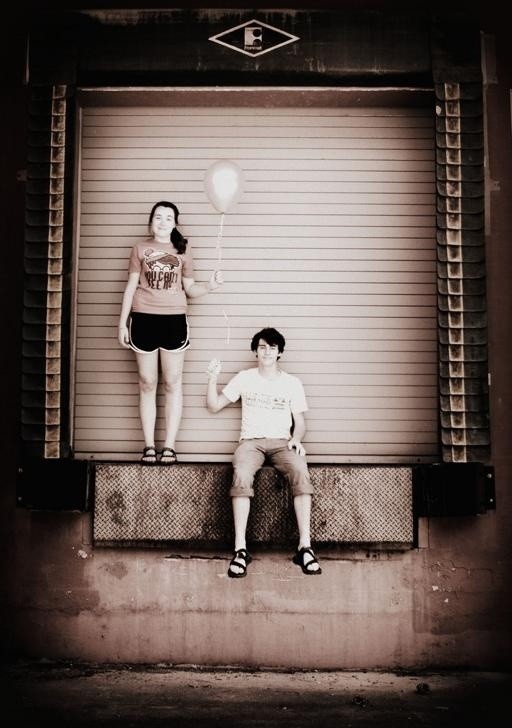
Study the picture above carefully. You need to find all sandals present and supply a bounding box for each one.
[226,548,253,579]
[291,546,322,576]
[158,446,179,465]
[140,445,158,466]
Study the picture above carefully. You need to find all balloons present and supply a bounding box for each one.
[204,160,246,216]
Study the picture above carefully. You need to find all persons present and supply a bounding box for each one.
[117,196,226,465]
[206,328,324,579]
